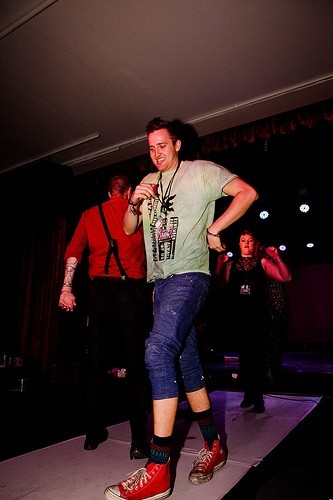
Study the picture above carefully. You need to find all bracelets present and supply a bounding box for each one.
[276,260,282,265]
[128,198,142,216]
[207,228,220,238]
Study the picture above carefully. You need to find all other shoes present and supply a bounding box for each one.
[84,431,108,450]
[130,445,151,460]
[247,407,265,414]
[240,400,253,408]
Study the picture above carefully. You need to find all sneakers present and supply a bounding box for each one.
[104,457,170,500]
[188,435,225,485]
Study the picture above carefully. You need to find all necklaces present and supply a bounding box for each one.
[240,259,254,295]
[155,162,182,240]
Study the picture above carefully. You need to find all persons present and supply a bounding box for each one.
[59,175,153,460]
[104,120,258,500]
[212,230,292,414]
